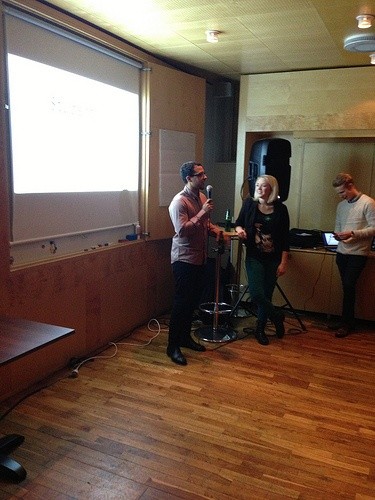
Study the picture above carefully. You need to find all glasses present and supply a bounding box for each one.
[190,172,207,177]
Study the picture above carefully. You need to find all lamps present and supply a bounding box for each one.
[356,14,374,29]
[205,30,220,43]
[369,53,375,64]
[344,33,375,53]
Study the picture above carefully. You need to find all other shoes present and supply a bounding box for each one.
[335,322,357,337]
[269,306,284,337]
[328,318,345,329]
[255,329,269,345]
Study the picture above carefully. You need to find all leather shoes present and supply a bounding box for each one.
[179,335,206,352]
[167,344,186,365]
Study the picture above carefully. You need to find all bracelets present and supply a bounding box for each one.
[351,231,354,237]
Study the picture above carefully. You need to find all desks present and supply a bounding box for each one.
[0,314,78,482]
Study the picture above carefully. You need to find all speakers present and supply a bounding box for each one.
[249,138,292,202]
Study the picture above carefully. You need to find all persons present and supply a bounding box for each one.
[166,162,226,366]
[234,175,290,345]
[332,173,375,337]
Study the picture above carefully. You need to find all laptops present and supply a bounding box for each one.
[322,231,339,252]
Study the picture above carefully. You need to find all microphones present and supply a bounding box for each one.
[206,185,213,218]
